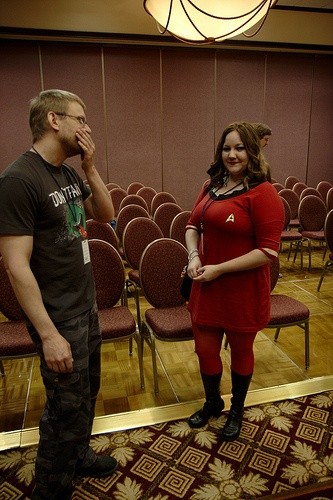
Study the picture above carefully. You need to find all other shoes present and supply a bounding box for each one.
[75,454,118,477]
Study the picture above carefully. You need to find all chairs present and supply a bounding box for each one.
[0,176,333,396]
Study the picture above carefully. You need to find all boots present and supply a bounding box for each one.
[220,368,253,441]
[189,370,223,428]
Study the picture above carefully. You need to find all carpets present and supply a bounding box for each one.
[0,390,333,500]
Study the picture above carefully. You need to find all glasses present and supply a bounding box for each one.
[55,112,89,128]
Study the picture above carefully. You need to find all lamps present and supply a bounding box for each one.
[143,0,279,46]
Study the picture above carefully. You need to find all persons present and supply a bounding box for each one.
[250,122,272,183]
[0,89,119,500]
[184,121,285,441]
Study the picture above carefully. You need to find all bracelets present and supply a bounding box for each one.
[187,249,199,263]
